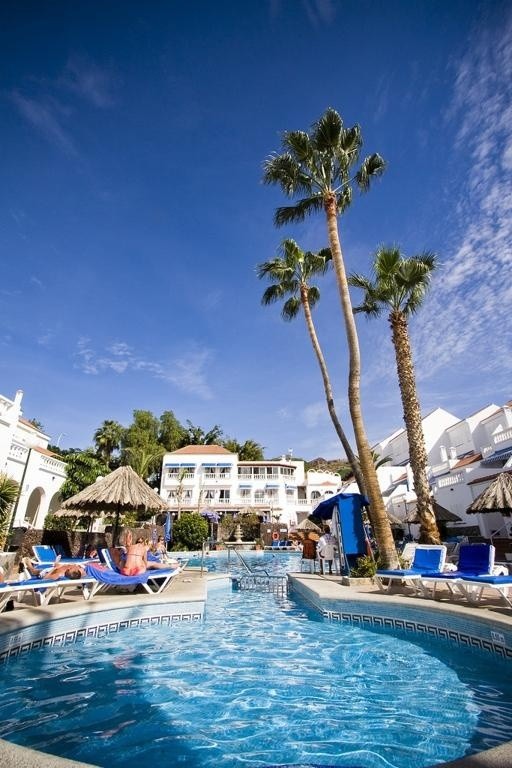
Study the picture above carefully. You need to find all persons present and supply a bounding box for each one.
[316,525,339,574]
[120,536,147,574]
[26,554,87,580]
[155,535,177,564]
[144,538,181,570]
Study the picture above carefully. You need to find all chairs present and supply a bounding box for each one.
[372,536,512,608]
[263,540,301,550]
[0,545,190,615]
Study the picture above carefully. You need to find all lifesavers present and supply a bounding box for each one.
[272,532,279,541]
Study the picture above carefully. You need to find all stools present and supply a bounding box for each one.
[300,557,338,575]
